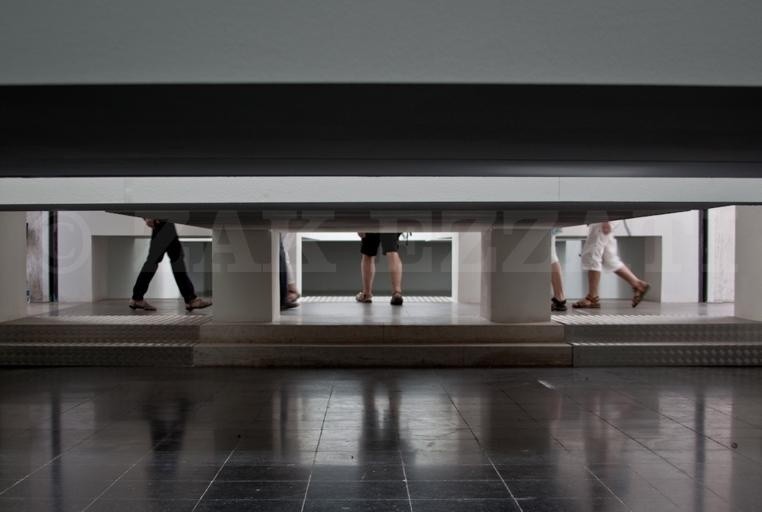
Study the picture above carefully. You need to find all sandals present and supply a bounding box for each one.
[572,295,599,308]
[391,292,402,305]
[551,298,567,311]
[357,292,372,302]
[632,280,650,308]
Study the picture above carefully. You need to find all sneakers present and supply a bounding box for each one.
[280,290,300,311]
[129,301,157,311]
[186,299,212,312]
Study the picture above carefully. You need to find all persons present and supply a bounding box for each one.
[128,215,213,312]
[280,231,301,311]
[280,231,300,302]
[551,232,570,312]
[571,218,652,309]
[354,230,407,304]
